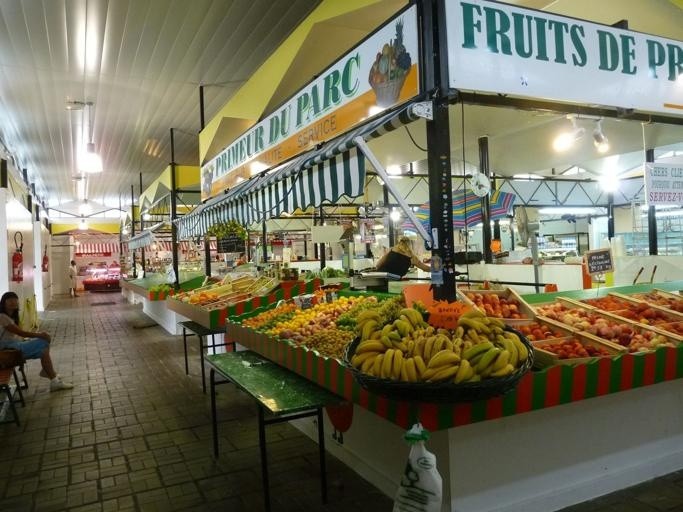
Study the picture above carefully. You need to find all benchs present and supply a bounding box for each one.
[0,351,33,432]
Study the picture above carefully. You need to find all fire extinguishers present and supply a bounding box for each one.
[11,231,23,281]
[42,244,49,272]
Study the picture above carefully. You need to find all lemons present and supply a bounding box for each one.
[382,43,390,56]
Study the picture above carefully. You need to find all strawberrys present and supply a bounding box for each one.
[397,49,412,70]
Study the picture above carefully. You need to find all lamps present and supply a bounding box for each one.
[588,115,612,157]
[547,115,583,150]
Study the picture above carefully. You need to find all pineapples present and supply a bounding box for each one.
[394,44,406,58]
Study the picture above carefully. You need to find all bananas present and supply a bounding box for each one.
[355,308,529,385]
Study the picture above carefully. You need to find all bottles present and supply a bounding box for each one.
[561,237,575,248]
[537,236,548,249]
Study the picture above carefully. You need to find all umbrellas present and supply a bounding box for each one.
[402,189,517,232]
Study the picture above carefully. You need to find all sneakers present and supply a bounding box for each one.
[50,377,74,392]
[38,368,61,380]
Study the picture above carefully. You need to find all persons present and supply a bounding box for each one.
[0,292,73,392]
[68,260,80,298]
[110,261,120,267]
[237,253,247,266]
[375,235,431,277]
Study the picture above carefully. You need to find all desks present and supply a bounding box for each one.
[175,317,226,395]
[198,348,343,511]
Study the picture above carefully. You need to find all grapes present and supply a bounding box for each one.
[305,294,406,360]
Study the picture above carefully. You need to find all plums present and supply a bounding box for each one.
[467,292,683,360]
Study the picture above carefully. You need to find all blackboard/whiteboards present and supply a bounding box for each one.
[584,247,615,276]
[217,229,245,253]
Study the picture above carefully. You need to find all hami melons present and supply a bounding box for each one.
[378,56,389,75]
